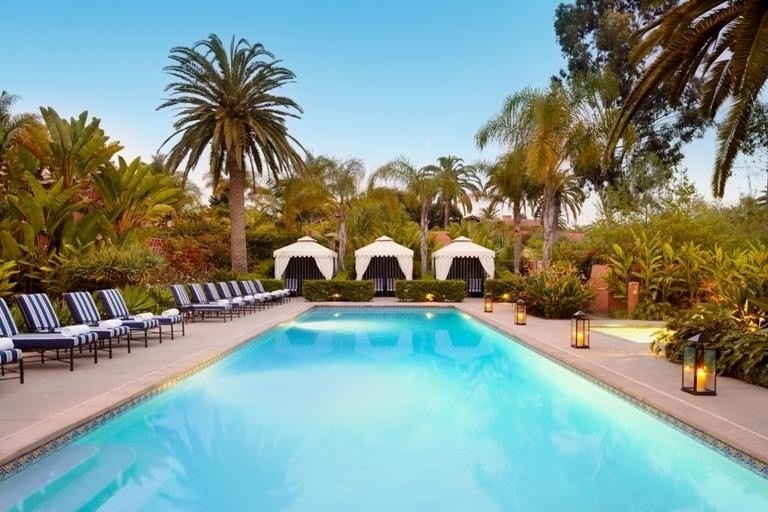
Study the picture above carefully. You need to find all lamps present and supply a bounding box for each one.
[484,292,493,312]
[570,309,590,349]
[681,333,718,396]
[514,298,527,325]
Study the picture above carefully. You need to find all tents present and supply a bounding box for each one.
[353,235,414,296]
[431,236,496,298]
[269,234,337,294]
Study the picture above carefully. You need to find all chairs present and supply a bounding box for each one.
[286,278,298,292]
[17,293,130,359]
[98,289,185,340]
[0,298,99,371]
[387,278,398,291]
[0,349,24,384]
[469,278,482,294]
[371,277,383,292]
[169,280,290,325]
[63,291,162,349]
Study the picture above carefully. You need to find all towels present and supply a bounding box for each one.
[0,337,14,351]
[61,324,91,337]
[134,313,154,322]
[162,307,180,317]
[97,318,123,329]
